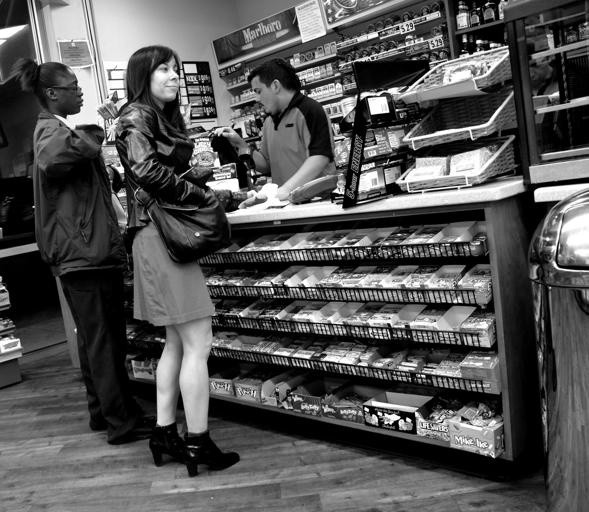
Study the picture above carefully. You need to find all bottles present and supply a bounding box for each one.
[566,26,578,44]
[456,0,506,56]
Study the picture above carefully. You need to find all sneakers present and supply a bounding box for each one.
[110,415,155,445]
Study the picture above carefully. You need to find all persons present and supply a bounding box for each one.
[209,56,338,201]
[115,43,242,478]
[12,55,159,446]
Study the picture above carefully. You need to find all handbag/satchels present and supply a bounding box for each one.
[150,190,232,264]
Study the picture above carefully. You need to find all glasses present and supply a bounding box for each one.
[51,85,78,92]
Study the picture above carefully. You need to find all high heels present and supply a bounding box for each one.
[150,423,185,466]
[183,432,240,477]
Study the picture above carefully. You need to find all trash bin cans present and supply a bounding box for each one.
[528,188,589,512]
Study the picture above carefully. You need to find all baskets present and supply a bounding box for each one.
[395,132,516,193]
[402,89,516,149]
[402,44,512,106]
